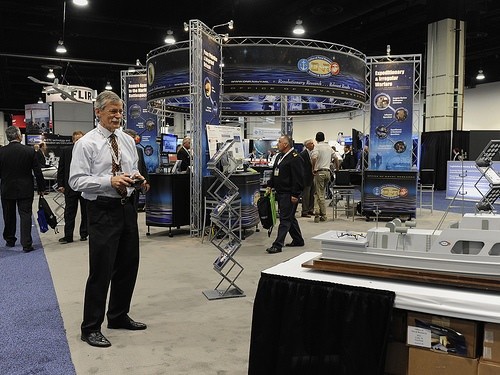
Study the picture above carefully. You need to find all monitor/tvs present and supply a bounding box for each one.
[161,133,178,153]
[25,134,43,146]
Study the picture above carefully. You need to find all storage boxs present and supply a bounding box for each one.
[387,308,500,375]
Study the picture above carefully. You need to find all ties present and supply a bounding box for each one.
[108,134,127,198]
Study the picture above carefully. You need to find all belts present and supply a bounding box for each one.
[87,198,133,205]
[318,169,330,171]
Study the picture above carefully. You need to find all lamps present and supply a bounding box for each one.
[183,22,189,32]
[164,30,176,44]
[212,20,234,31]
[46,68,55,79]
[55,1,67,54]
[475,70,484,80]
[105,81,112,90]
[218,33,229,41]
[293,19,305,35]
[37,97,43,103]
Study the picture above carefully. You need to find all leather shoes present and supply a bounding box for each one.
[81,331,112,347]
[107,318,147,330]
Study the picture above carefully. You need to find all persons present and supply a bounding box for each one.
[0,126,46,252]
[33,142,50,168]
[124,129,149,210]
[266,151,273,161]
[57,130,88,243]
[360,146,368,170]
[326,145,358,199]
[310,132,338,222]
[68,90,147,348]
[453,148,461,161]
[177,138,191,171]
[300,139,315,218]
[265,134,307,252]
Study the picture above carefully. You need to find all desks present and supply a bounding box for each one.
[144,170,261,237]
[246,251,500,375]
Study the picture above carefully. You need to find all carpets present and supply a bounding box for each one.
[1,204,77,375]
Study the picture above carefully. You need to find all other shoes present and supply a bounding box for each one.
[5,243,15,247]
[320,216,326,221]
[285,241,296,247]
[308,211,315,215]
[23,247,34,253]
[266,246,282,253]
[301,213,311,218]
[79,237,87,241]
[314,217,320,223]
[59,236,73,243]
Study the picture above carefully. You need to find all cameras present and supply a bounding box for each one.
[130,178,144,187]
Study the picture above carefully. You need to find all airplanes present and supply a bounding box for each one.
[27,76,80,102]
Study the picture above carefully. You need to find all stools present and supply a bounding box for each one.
[200,195,242,243]
[417,168,435,214]
[331,171,356,222]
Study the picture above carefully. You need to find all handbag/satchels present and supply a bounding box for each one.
[257,190,276,237]
[37,195,49,233]
[40,195,58,229]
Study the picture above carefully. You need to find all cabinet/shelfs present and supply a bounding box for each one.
[201,139,245,300]
